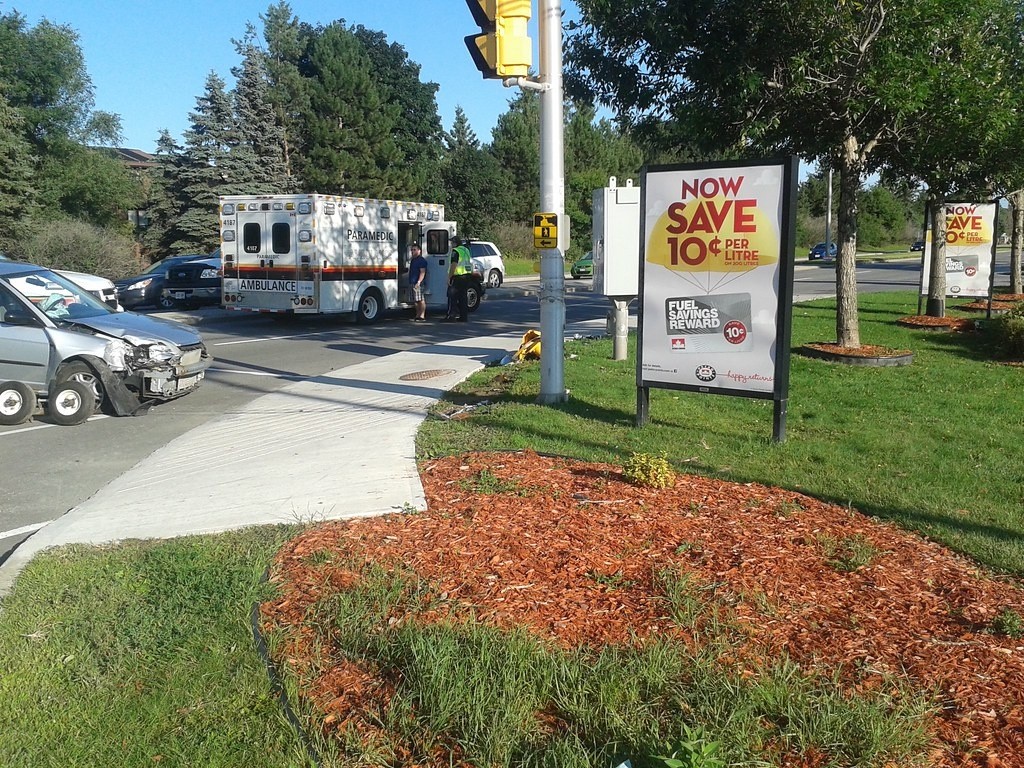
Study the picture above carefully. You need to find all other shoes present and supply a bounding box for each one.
[415,317,426,322]
[438,316,456,323]
[456,318,467,322]
[409,318,415,321]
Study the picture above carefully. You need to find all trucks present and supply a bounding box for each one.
[218,193,489,326]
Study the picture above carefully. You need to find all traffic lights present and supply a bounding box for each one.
[464,0,532,77]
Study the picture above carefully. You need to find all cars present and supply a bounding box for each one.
[110,255,210,310]
[0,258,214,427]
[458,237,505,289]
[570,250,593,280]
[809,241,837,261]
[910,241,924,252]
[0,254,124,315]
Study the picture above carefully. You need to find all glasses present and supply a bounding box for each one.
[411,249,419,251]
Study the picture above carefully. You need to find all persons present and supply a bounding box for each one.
[440,236,473,322]
[406,244,428,322]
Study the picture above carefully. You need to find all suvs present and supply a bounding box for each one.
[166,246,221,311]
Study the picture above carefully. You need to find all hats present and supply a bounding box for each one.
[448,236,462,246]
